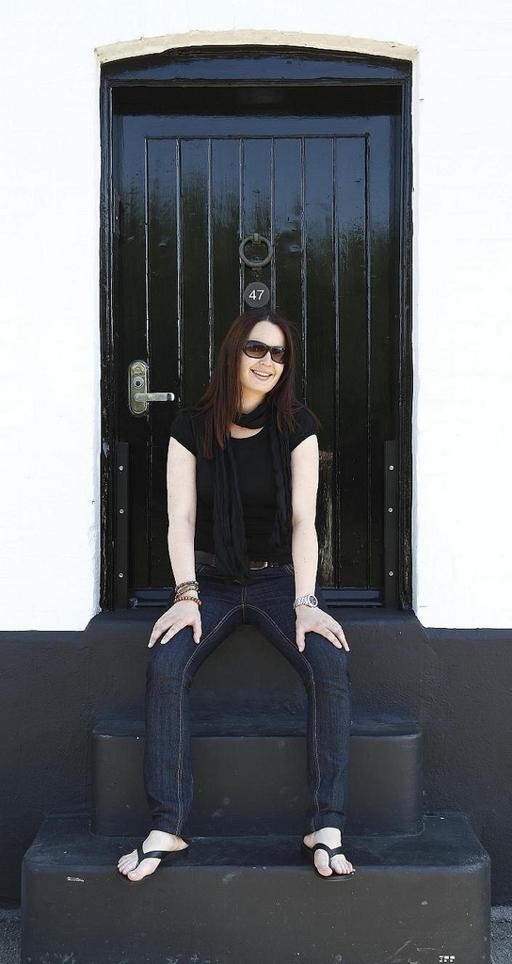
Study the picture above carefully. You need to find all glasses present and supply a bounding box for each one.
[241,340,288,364]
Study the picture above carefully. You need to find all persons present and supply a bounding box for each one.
[115,308,354,883]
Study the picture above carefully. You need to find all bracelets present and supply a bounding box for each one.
[173,581,203,605]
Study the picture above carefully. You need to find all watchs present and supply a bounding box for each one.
[293,594,318,609]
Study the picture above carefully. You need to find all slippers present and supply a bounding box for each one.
[302,836,354,883]
[113,837,191,884]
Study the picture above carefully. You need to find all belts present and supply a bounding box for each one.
[195,551,279,571]
